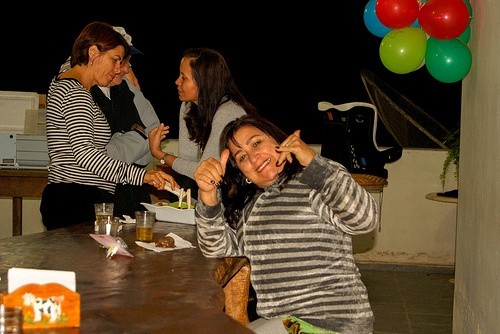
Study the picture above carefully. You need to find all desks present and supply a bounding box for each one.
[0,169,50,236]
[425,192,458,284]
[0,219,257,334]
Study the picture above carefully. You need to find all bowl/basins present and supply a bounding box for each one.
[140,202,196,226]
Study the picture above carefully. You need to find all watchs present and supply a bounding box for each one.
[160,152,169,165]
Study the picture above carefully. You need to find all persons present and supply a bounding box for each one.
[194,114,380,334]
[38,21,180,231]
[149,48,259,211]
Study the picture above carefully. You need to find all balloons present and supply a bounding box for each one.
[363,0,473,84]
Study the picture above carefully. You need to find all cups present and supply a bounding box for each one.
[98,215,119,248]
[135,212,155,241]
[94,203,115,225]
[0,307,23,334]
